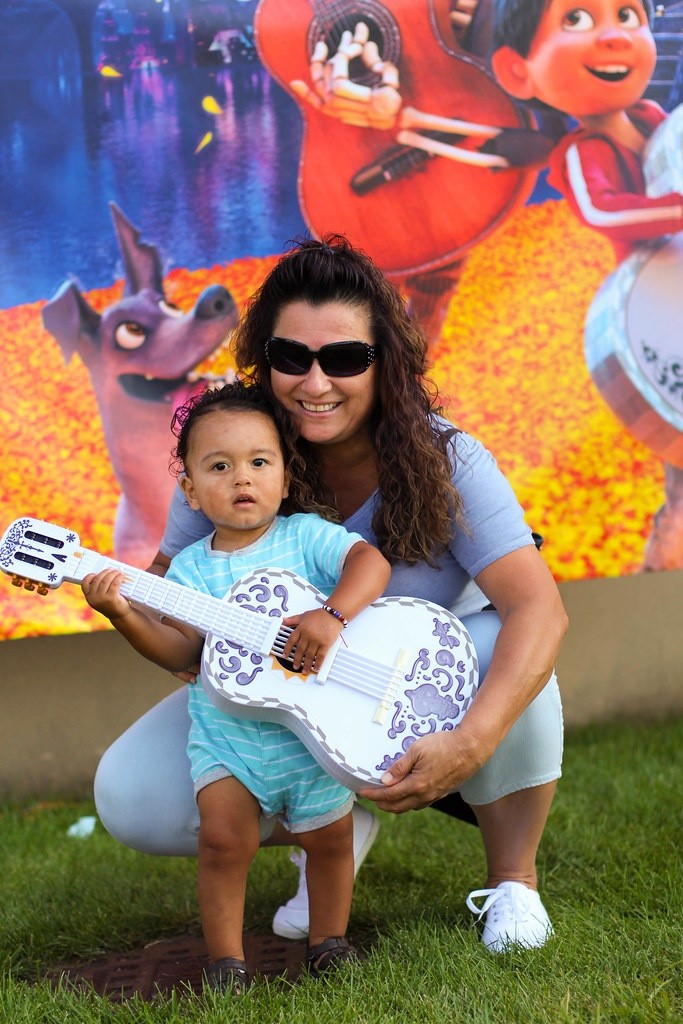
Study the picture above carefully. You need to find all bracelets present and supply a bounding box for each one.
[321,605,348,630]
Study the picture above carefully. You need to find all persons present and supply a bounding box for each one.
[94,232,569,958]
[81,381,391,996]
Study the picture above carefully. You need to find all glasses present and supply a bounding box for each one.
[264,336,378,376]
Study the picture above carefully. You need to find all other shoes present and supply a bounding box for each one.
[204,958,250,1000]
[305,939,364,983]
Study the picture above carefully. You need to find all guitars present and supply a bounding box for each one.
[1,516,481,792]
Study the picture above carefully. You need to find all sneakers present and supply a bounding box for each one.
[466,882,557,952]
[272,803,380,939]
